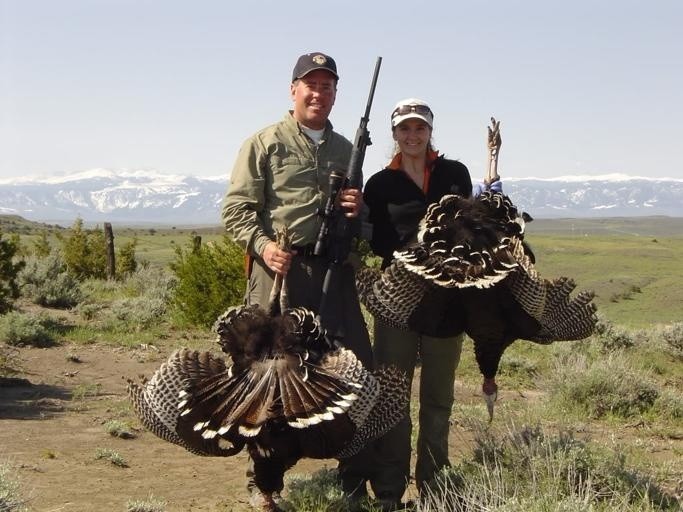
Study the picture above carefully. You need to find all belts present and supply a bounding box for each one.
[290,243,328,258]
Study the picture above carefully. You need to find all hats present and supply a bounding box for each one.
[291,52,340,82]
[391,95,435,129]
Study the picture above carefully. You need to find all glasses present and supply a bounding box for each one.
[391,103,435,123]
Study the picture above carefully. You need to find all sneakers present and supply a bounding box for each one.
[248,487,285,512]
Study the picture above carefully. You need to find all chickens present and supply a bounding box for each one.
[356,115,600,423]
[125,221,412,512]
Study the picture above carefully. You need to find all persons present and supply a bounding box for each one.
[364,99,472,512]
[221,52,372,512]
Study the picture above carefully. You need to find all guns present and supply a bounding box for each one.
[311,58,381,336]
